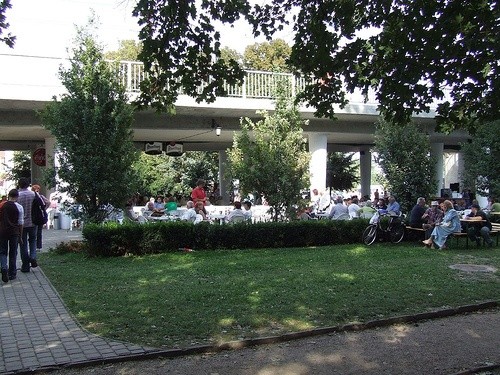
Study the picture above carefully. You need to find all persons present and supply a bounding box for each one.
[462,189,500,223]
[423,194,461,250]
[31,185,51,251]
[465,204,494,247]
[409,197,428,243]
[15,177,45,273]
[104,196,252,224]
[0,189,24,283]
[286,189,401,221]
[192,179,209,208]
[51,196,85,229]
[421,201,444,249]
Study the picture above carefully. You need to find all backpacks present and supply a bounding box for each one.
[31,192,48,225]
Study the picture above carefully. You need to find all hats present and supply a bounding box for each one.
[430,201,439,205]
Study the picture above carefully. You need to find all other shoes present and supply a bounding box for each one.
[1,268,8,282]
[21,268,30,272]
[476,237,481,247]
[487,241,493,248]
[422,239,433,244]
[438,244,447,250]
[31,256,37,268]
[9,275,16,280]
[422,223,430,230]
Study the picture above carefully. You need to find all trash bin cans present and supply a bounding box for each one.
[54,213,60,230]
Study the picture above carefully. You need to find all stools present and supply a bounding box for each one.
[491,222,500,246]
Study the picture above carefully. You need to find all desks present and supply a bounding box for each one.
[148,215,178,220]
[207,213,226,221]
[318,211,327,220]
[490,212,500,220]
[460,219,487,246]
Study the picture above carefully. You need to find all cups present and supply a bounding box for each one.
[435,220,439,225]
[422,223,427,231]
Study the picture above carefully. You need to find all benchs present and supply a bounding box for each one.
[407,227,467,249]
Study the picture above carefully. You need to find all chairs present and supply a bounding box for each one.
[136,208,352,221]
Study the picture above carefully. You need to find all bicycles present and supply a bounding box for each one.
[362,206,405,246]
